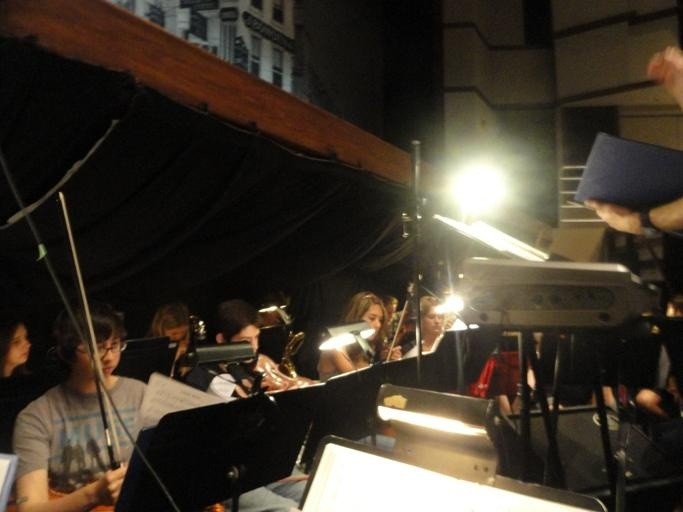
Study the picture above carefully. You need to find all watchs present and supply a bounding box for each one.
[640,208,666,242]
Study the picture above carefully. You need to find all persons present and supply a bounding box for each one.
[186,300,308,512]
[318,292,401,381]
[594,296,683,430]
[149,304,197,381]
[259,292,319,381]
[385,297,401,341]
[585,45,683,243]
[13,304,146,511]
[0,316,52,456]
[400,296,456,359]
[476,333,561,433]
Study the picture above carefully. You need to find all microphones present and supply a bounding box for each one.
[401,213,408,240]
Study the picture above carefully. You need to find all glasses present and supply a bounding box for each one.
[75,338,128,355]
